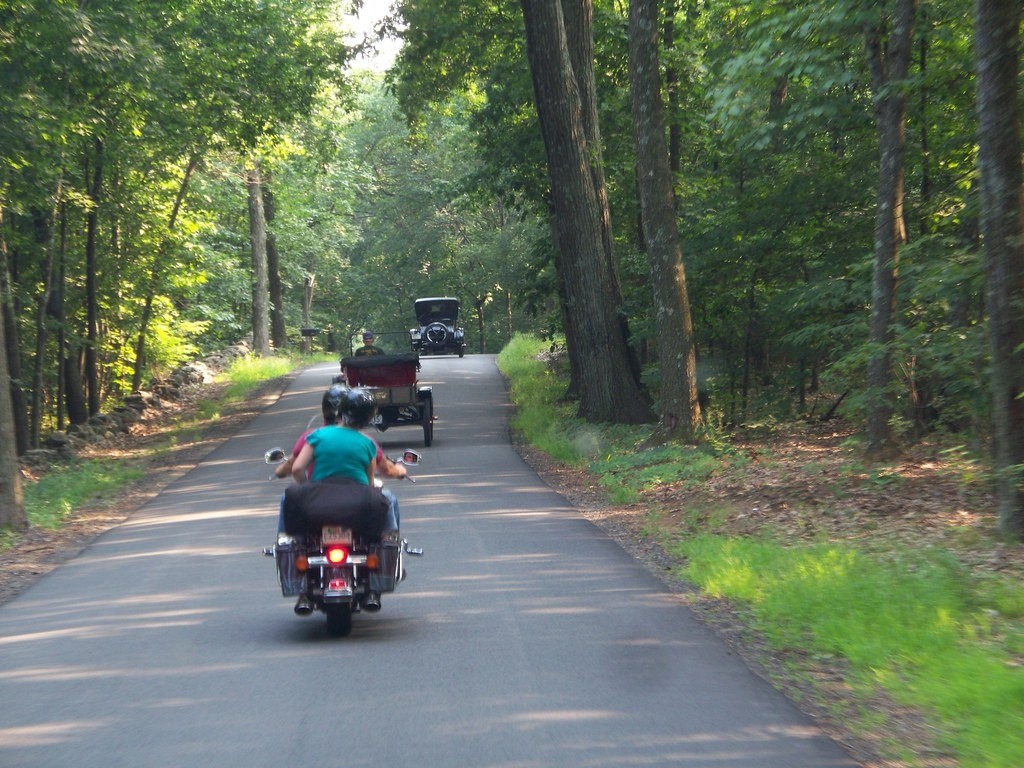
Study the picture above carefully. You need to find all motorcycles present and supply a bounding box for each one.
[263,413,425,639]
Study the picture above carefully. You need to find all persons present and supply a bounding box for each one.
[275,387,408,542]
[404,451,417,464]
[354,332,385,357]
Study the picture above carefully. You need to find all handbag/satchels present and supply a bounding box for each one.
[283,478,391,534]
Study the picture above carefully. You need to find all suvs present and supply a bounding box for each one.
[404,297,466,358]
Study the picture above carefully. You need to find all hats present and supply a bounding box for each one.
[363,331,373,341]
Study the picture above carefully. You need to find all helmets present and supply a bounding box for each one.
[339,386,377,427]
[322,384,352,423]
[270,450,283,461]
[405,450,419,463]
